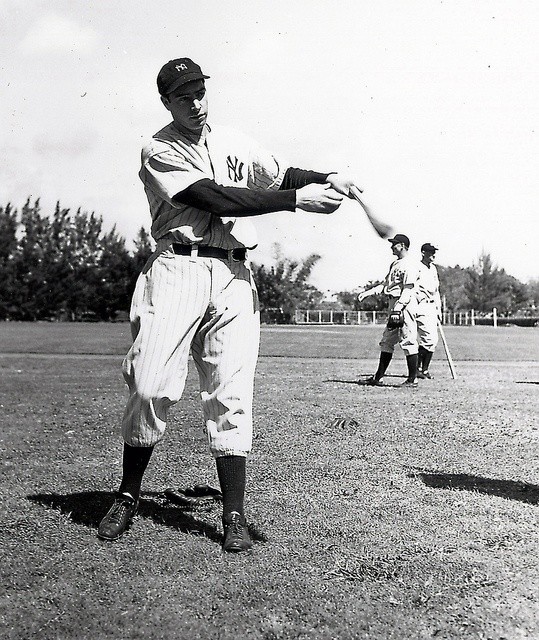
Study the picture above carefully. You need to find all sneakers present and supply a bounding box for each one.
[394,378,418,387]
[222,514,252,553]
[96,490,139,542]
[358,376,384,385]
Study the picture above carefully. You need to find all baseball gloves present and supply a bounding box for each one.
[388,311,403,328]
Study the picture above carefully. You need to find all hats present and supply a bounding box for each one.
[421,243,438,251]
[157,57,210,96]
[388,234,410,248]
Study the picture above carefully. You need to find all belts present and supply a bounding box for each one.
[172,243,248,262]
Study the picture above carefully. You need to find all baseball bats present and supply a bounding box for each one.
[438,319,457,378]
[350,184,393,237]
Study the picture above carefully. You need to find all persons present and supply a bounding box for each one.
[96,58,364,555]
[417,243,442,380]
[357,234,421,387]
